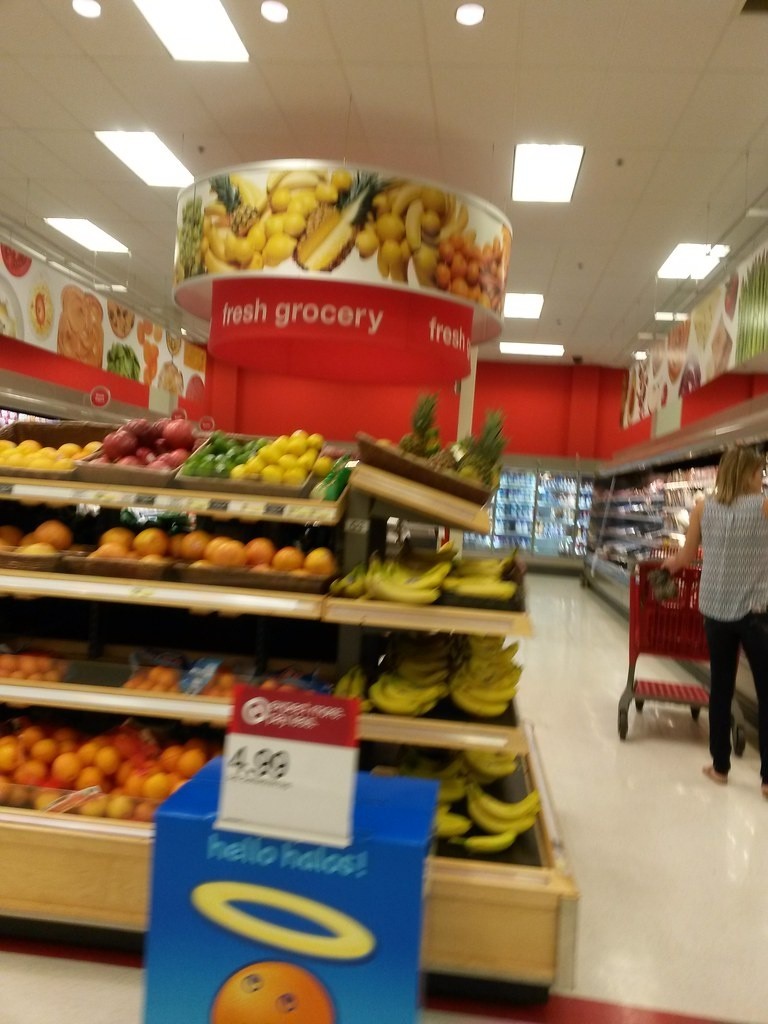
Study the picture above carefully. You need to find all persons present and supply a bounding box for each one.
[657,442,768,801]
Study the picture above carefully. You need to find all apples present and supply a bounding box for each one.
[104,415,205,471]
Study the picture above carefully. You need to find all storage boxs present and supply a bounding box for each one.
[175,431,329,498]
[76,427,216,487]
[0,421,123,482]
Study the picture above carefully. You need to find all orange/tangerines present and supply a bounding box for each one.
[438,236,502,316]
[1,654,317,707]
[185,430,268,475]
[0,718,225,821]
[0,522,336,579]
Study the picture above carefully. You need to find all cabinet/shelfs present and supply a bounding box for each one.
[1,466,569,989]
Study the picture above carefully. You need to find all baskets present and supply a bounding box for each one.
[62,556,176,581]
[173,561,341,595]
[0,545,97,573]
[175,431,327,499]
[74,430,214,488]
[355,430,489,506]
[0,420,120,481]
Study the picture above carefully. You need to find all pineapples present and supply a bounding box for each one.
[212,175,260,234]
[398,390,510,488]
[179,199,202,278]
[295,174,384,272]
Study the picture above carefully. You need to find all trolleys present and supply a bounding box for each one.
[616,544,748,758]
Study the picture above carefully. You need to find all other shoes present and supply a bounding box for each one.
[761,784,768,798]
[702,765,728,786]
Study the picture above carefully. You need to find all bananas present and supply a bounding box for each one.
[367,744,541,854]
[328,537,519,605]
[388,190,474,287]
[332,632,521,715]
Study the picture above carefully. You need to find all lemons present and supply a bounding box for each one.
[232,431,331,494]
[206,171,407,277]
[0,438,101,472]
